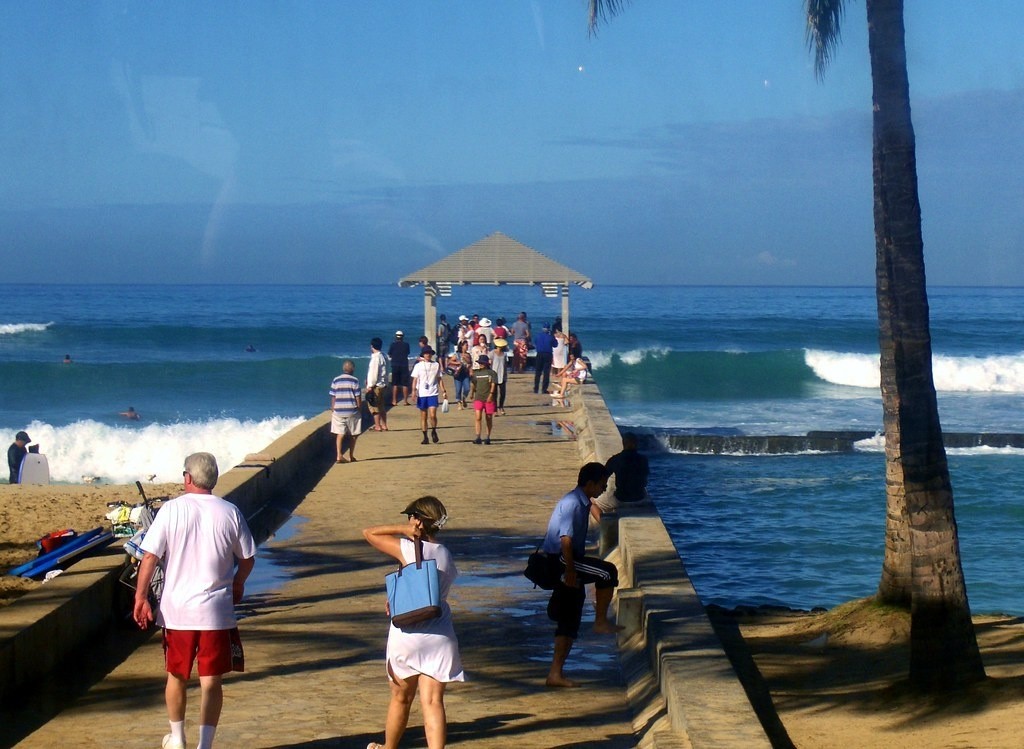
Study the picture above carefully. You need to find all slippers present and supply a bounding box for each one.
[404,403,411,406]
[369,426,388,432]
[336,457,357,463]
[390,403,397,406]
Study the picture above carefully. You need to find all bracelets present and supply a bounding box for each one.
[490,392,494,394]
[443,391,446,393]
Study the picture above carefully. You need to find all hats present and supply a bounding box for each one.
[420,345,435,357]
[364,390,379,408]
[543,322,550,329]
[400,507,429,523]
[458,315,507,326]
[396,330,403,337]
[16,431,31,443]
[476,355,491,365]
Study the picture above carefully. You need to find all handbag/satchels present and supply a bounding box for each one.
[385,535,442,628]
[446,352,463,378]
[523,551,560,590]
[571,370,580,378]
[442,393,449,412]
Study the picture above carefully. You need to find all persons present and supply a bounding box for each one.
[363,336,389,432]
[329,361,361,463]
[8,431,31,484]
[64,354,72,362]
[541,462,625,688]
[119,407,138,419]
[590,432,650,523]
[133,452,257,749]
[362,496,469,749]
[416,312,589,416]
[468,355,498,444]
[388,331,412,405]
[411,345,447,444]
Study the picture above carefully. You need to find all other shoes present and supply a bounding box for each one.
[432,432,438,443]
[500,408,506,415]
[421,438,429,444]
[494,412,498,417]
[473,439,491,444]
[457,400,467,411]
[162,734,186,749]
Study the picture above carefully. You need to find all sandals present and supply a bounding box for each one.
[367,742,383,749]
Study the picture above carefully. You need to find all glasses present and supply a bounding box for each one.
[183,471,191,476]
[599,482,607,488]
[408,515,413,521]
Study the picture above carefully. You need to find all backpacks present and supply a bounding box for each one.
[440,323,461,346]
